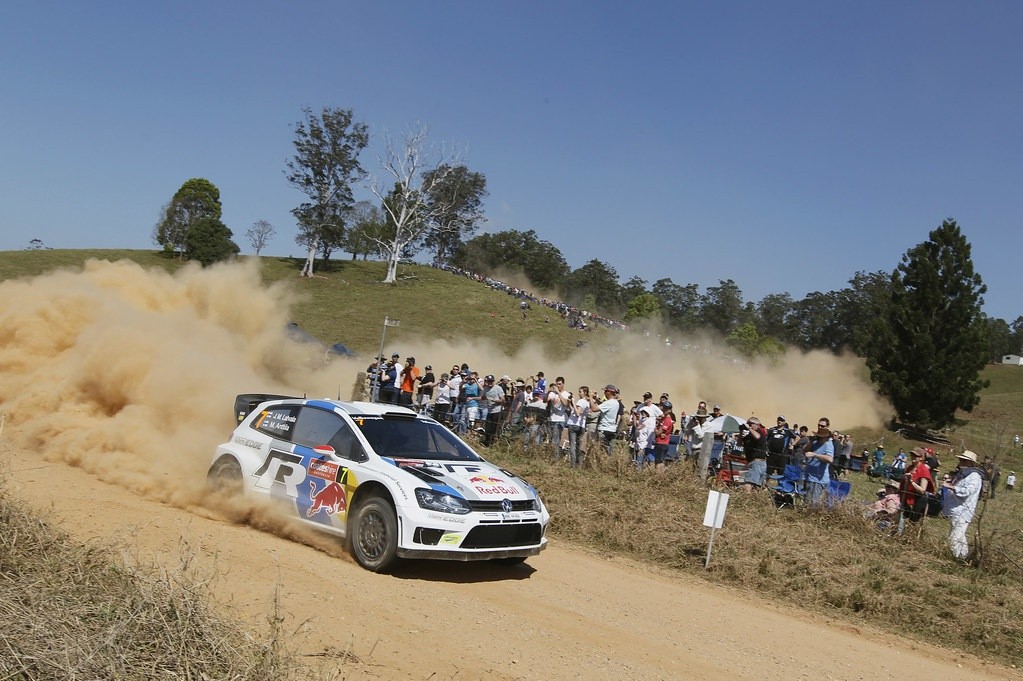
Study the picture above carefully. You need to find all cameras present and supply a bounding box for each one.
[467,378,475,381]
[568,392,573,399]
[739,425,749,430]
[693,417,697,422]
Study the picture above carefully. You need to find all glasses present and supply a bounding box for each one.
[818,424,826,427]
[885,486,891,489]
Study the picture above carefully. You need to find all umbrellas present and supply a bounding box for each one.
[708,414,747,433]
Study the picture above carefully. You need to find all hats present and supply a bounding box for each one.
[682,412,686,418]
[661,402,672,409]
[956,450,979,464]
[877,445,884,449]
[638,406,650,417]
[535,371,545,377]
[695,410,710,418]
[377,352,511,386]
[714,403,721,410]
[601,385,617,392]
[909,448,925,457]
[884,480,901,490]
[778,415,786,421]
[514,382,525,386]
[643,392,652,398]
[634,401,642,405]
[661,393,669,399]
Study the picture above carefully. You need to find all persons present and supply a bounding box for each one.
[943,451,982,557]
[944,434,1023,500]
[367,355,942,535]
[433,263,748,366]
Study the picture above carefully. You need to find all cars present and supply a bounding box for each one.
[202,393,553,575]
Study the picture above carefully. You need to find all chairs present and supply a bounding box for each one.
[766,464,804,509]
[708,441,726,477]
[826,480,851,508]
[664,435,682,461]
